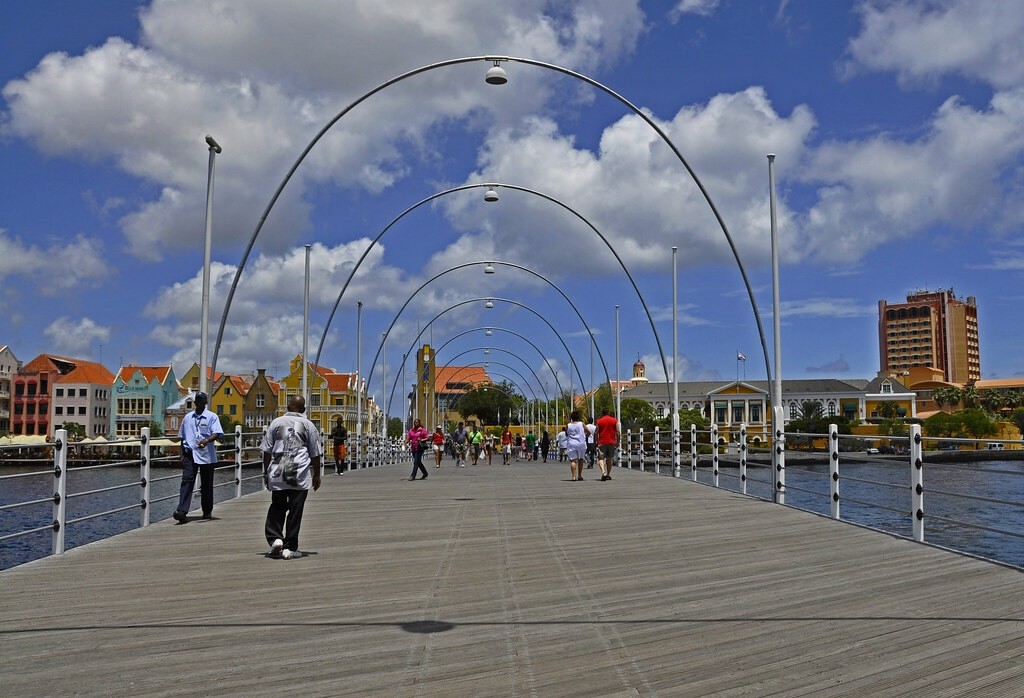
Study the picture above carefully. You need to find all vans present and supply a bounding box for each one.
[988,443,1005,451]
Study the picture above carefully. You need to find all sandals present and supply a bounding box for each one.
[601,474,608,481]
[607,475,612,480]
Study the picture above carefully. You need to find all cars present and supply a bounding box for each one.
[941,445,959,450]
[866,445,912,455]
[619,448,672,457]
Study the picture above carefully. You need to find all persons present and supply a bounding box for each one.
[173,392,224,524]
[259,395,322,560]
[594,407,620,481]
[556,427,568,462]
[441,430,539,462]
[430,424,445,468]
[565,411,592,481]
[404,419,429,481]
[585,417,597,469]
[500,425,513,465]
[482,428,495,465]
[450,421,470,468]
[0,449,250,460]
[468,426,483,465]
[330,418,347,476]
[541,431,550,463]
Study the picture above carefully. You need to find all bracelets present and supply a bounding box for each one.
[205,439,209,443]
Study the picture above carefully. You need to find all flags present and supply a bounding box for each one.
[737,352,746,362]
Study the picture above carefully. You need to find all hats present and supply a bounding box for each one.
[196,392,207,401]
[437,425,442,428]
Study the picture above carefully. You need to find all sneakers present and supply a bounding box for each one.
[268,539,283,556]
[281,549,302,559]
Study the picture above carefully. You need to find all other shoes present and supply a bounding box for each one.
[173,511,187,523]
[203,512,211,519]
[578,477,583,481]
[408,476,415,481]
[336,470,344,476]
[420,474,428,479]
[507,462,510,464]
[456,461,459,466]
[437,465,439,468]
[462,463,465,468]
[572,478,576,481]
[503,462,506,465]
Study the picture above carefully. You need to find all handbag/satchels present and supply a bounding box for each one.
[479,450,485,460]
[418,440,428,450]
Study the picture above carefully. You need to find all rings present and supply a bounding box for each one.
[201,444,204,447]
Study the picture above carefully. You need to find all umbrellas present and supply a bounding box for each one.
[0,434,224,446]
